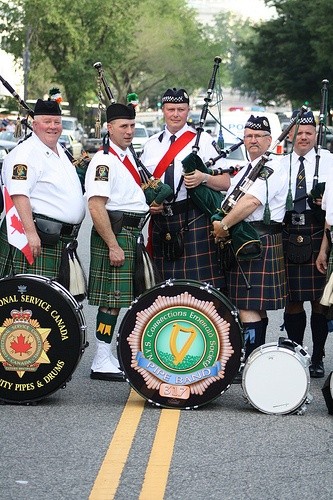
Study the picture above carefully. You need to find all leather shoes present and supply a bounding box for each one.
[309,358,324,377]
[90,369,127,381]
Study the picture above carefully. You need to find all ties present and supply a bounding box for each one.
[294,156,305,214]
[223,163,252,208]
[164,135,176,202]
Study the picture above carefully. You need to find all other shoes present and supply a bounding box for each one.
[233,367,246,383]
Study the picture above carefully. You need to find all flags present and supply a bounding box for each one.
[3,187,34,265]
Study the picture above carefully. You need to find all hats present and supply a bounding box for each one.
[161,87,188,105]
[290,109,317,127]
[106,94,138,123]
[34,88,61,115]
[244,114,270,133]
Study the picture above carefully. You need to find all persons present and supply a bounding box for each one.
[5,120,15,132]
[210,115,288,379]
[281,109,333,398]
[140,88,230,293]
[0,100,84,290]
[84,104,163,380]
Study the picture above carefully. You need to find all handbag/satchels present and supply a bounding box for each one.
[161,231,185,259]
[288,234,312,264]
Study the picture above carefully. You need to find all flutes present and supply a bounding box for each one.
[168,55,244,232]
[0,74,79,166]
[312,79,330,197]
[92,62,175,217]
[215,104,308,221]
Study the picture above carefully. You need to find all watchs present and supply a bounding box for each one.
[202,174,208,185]
[221,221,228,230]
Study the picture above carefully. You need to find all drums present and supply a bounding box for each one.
[115,279,249,411]
[240,337,313,416]
[0,273,89,406]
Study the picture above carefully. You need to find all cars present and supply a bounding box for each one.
[0,105,284,166]
